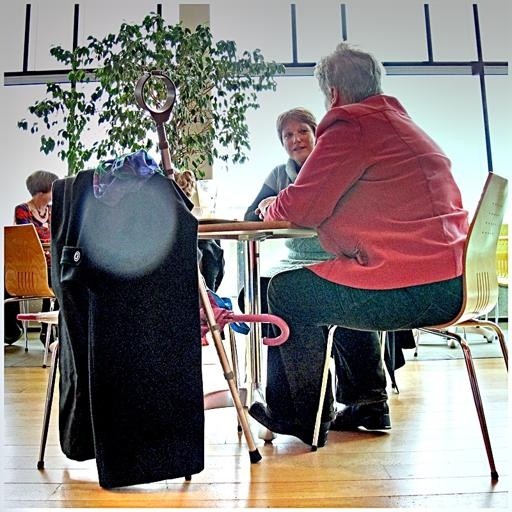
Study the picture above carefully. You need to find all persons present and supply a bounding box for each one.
[246,41,471,448]
[236,105,319,340]
[14,168,61,353]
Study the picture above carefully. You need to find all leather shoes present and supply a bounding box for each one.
[248,401,329,447]
[329,401,390,431]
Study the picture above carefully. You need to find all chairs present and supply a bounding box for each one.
[4,223,57,368]
[34,171,265,482]
[311,172,508,479]
[485,223,508,326]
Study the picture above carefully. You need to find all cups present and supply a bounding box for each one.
[196,178,219,220]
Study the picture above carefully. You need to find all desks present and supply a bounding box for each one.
[196,220,320,462]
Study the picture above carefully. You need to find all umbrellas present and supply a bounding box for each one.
[199,286,291,348]
